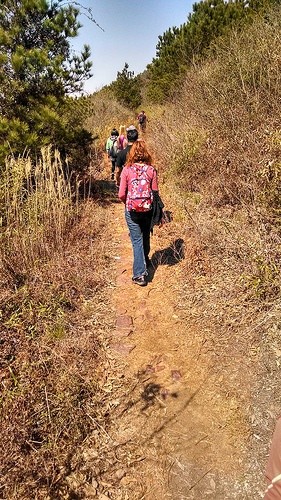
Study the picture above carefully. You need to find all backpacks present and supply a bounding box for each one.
[139,115,145,124]
[125,163,153,212]
[109,137,119,158]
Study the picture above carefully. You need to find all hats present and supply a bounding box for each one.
[126,125,136,132]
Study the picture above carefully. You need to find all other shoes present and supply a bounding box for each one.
[111,173,114,180]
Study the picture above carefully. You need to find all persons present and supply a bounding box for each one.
[107,123,139,190]
[118,139,159,286]
[138,110,147,134]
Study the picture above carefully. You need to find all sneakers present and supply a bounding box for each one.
[132,276,147,286]
